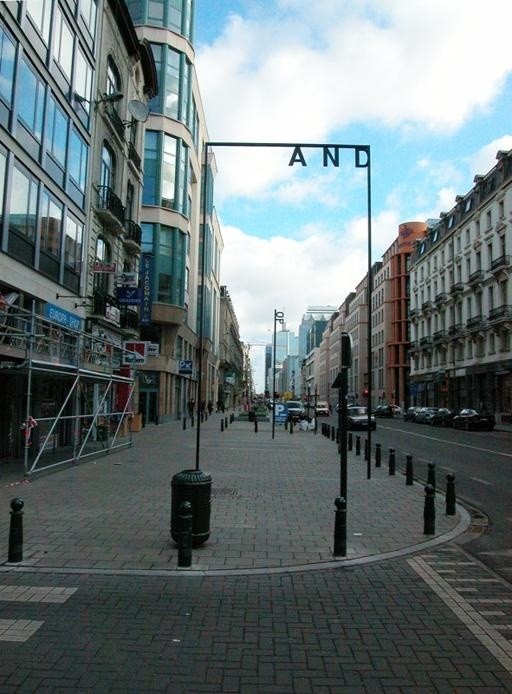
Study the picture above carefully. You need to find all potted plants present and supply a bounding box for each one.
[102,257,115,271]
[91,256,102,271]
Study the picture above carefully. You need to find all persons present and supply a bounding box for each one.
[187,398,195,416]
[215,398,225,414]
[207,400,214,415]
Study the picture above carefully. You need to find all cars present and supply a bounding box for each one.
[287,400,305,416]
[443,408,495,430]
[377,404,451,425]
[347,406,376,430]
[316,401,330,416]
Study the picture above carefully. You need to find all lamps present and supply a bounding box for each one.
[128,99,152,129]
[93,92,124,111]
[56,290,104,300]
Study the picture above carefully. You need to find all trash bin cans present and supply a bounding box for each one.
[98,424,110,441]
[170,469,213,548]
